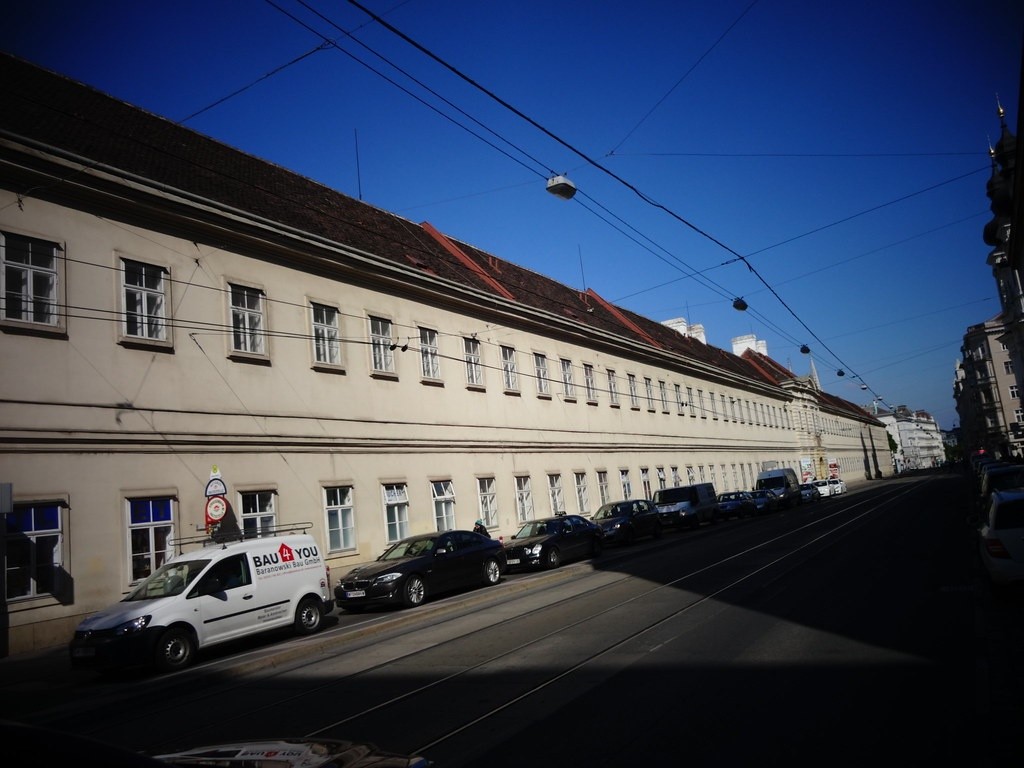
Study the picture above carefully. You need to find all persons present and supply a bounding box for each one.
[473,520,491,538]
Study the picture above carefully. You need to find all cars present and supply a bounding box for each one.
[593,500,661,545]
[501,515,605,572]
[969,447,1024,593]
[716,491,759,518]
[812,480,836,499]
[800,482,821,505]
[335,529,507,612]
[750,489,779,513]
[830,479,848,495]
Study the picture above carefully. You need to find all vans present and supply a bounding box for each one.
[654,483,719,531]
[70,523,334,669]
[752,469,801,511]
